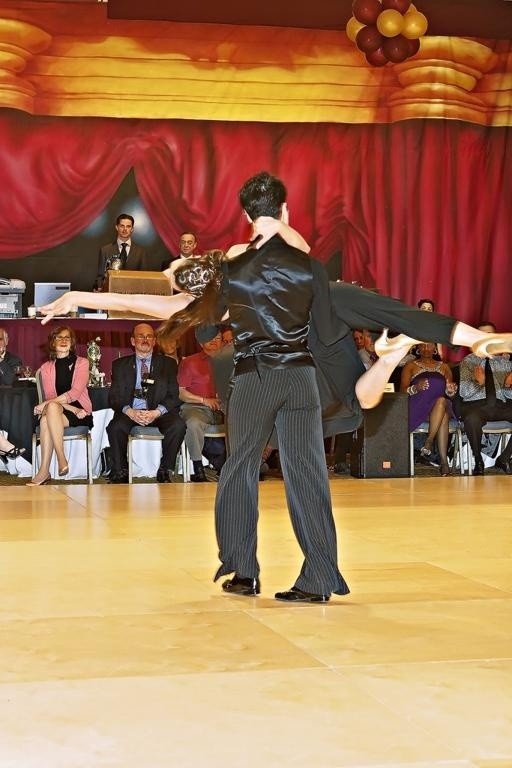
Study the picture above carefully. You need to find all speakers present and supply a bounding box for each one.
[350,393,409,478]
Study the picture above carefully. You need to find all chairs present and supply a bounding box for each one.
[468,420,512,477]
[124,425,187,485]
[188,425,227,484]
[409,419,464,475]
[36,367,93,487]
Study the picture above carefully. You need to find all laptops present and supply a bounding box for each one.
[34,283,71,318]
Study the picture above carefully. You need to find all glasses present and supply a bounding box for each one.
[134,334,155,340]
[54,336,72,340]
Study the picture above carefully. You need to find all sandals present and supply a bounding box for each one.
[59,462,69,476]
[439,463,452,477]
[25,474,51,487]
[421,439,432,458]
[0,445,27,464]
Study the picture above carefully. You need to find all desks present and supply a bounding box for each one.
[3,316,232,377]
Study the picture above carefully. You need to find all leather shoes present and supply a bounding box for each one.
[222,576,260,596]
[112,471,126,483]
[274,586,329,602]
[496,456,512,475]
[474,458,483,474]
[194,461,206,481]
[156,469,171,483]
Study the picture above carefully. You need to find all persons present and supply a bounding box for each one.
[178,330,222,482]
[160,232,200,273]
[400,341,458,478]
[212,171,350,603]
[222,328,233,347]
[0,327,23,389]
[93,214,146,293]
[106,323,187,483]
[25,326,94,486]
[0,430,26,465]
[459,321,512,476]
[159,338,179,364]
[36,217,512,449]
[353,330,377,370]
[418,298,434,312]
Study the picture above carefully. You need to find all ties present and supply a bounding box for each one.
[485,357,496,407]
[141,358,148,378]
[121,243,128,267]
[370,354,385,408]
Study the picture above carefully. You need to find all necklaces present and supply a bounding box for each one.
[414,360,443,370]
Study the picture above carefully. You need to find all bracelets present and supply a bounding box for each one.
[406,385,417,396]
[445,389,455,398]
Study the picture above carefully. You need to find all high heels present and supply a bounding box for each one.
[374,327,430,356]
[471,333,512,360]
